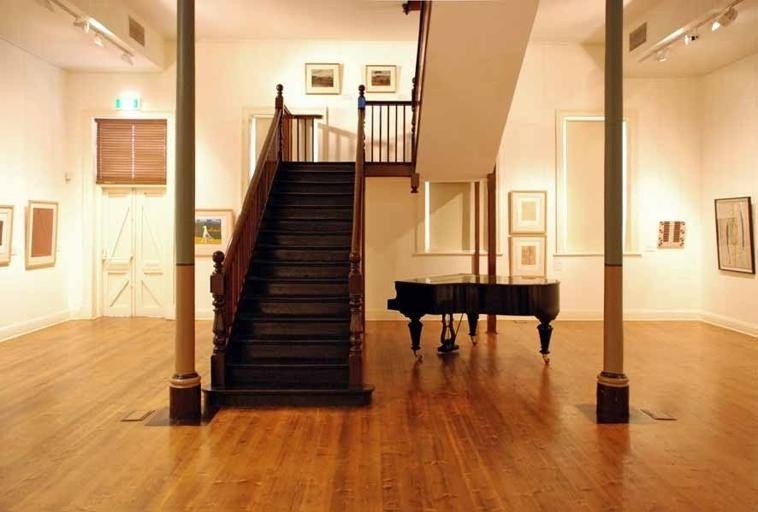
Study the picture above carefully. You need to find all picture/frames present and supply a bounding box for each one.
[26,199,59,269]
[0,204,15,265]
[305,62,340,95]
[365,65,397,93]
[195,208,236,257]
[510,236,547,276]
[510,190,548,234]
[714,196,755,274]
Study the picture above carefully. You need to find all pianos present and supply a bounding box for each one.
[387,274,560,364]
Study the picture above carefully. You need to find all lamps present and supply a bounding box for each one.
[45,0,136,65]
[639,0,744,63]
